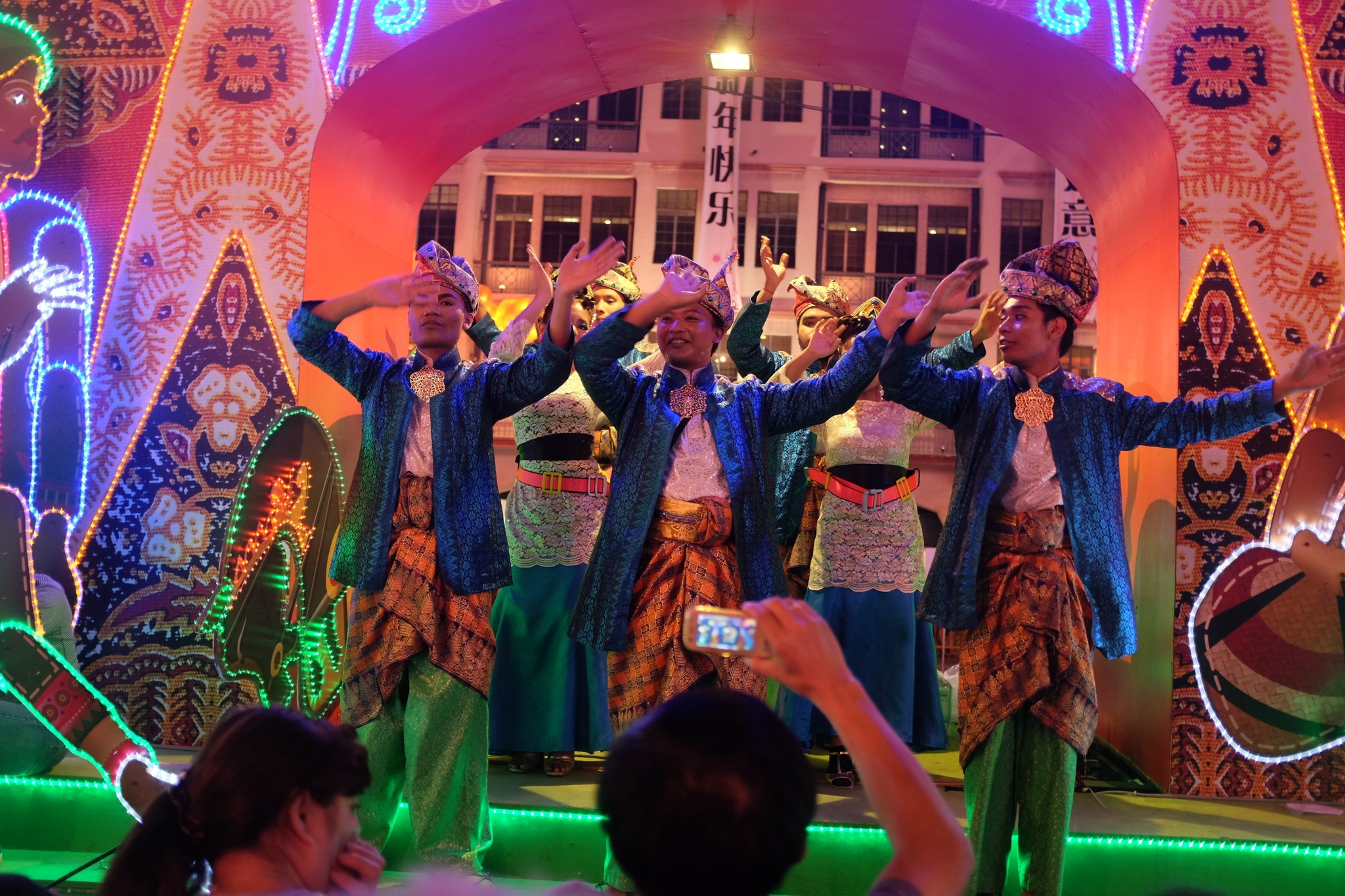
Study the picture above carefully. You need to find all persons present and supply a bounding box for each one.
[589,595,972,895]
[485,241,668,776]
[725,232,1009,599]
[465,253,654,485]
[572,253,933,745]
[765,313,950,790]
[875,237,1345,895]
[101,705,387,896]
[286,235,630,868]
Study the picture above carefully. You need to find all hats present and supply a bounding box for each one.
[661,250,739,331]
[410,240,479,318]
[590,255,644,302]
[786,275,851,323]
[999,241,1099,331]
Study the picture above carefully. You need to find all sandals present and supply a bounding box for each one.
[544,755,575,776]
[508,753,544,773]
[825,752,854,789]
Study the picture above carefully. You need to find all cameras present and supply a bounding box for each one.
[682,604,772,660]
